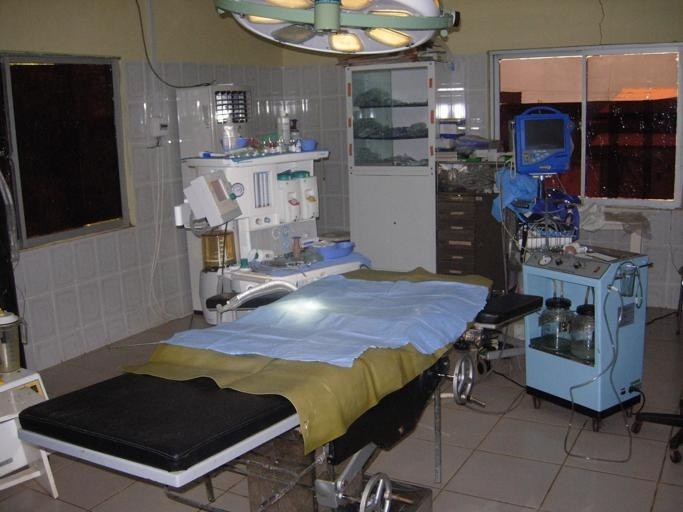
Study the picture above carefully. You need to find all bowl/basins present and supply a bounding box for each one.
[302,139,317,151]
[220,138,249,152]
[317,241,356,259]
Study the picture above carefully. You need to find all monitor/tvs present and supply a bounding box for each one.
[517,115,570,155]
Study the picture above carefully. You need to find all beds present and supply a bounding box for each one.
[15,274,549,512]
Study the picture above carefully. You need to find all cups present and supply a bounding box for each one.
[620,264,636,297]
[0,312,28,374]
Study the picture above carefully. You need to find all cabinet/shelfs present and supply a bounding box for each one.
[347,66,442,277]
[438,154,518,298]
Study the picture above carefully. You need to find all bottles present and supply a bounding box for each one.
[541,296,596,360]
[288,118,302,153]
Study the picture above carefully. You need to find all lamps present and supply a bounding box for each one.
[212,0,465,64]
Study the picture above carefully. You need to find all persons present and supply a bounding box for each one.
[596,132,633,198]
